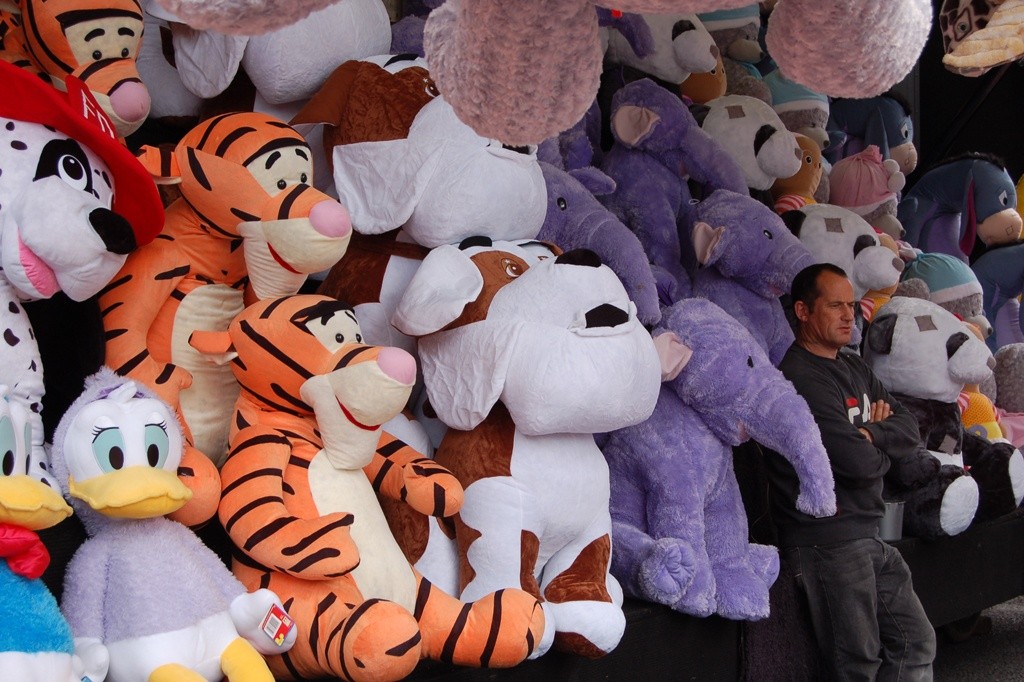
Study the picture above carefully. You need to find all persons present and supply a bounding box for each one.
[775,262,944,681]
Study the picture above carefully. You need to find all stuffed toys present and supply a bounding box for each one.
[802,0,1024,629]
[0,0,800,682]
